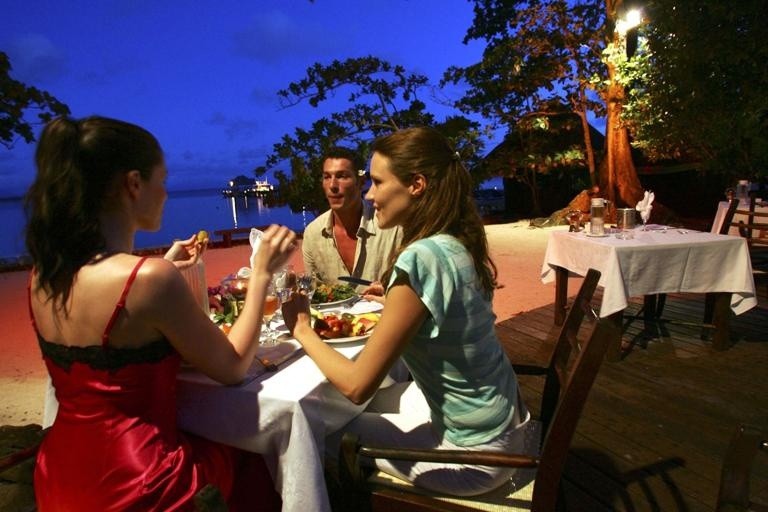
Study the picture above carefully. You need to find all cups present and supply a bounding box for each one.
[262,283,281,347]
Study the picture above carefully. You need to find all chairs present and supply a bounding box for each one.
[346,268,618,512]
[656,198,768,323]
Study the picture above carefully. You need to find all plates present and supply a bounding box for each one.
[309,314,382,342]
[307,289,356,305]
[317,301,385,315]
[353,299,356,301]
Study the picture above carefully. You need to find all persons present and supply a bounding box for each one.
[301,145,404,295]
[27,114,299,512]
[281,127,532,499]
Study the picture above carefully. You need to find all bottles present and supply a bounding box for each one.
[590,198,610,236]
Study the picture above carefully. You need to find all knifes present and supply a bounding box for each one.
[343,293,365,308]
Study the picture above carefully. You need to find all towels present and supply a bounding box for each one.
[635,190,654,221]
[249,228,286,290]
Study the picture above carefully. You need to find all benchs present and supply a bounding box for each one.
[215,224,281,247]
